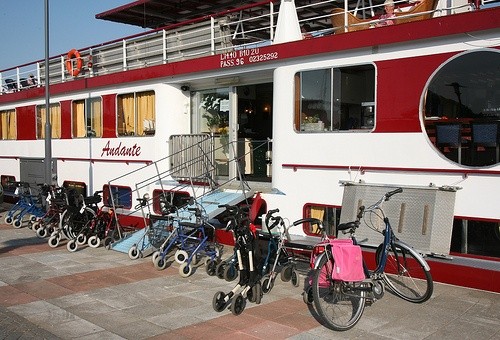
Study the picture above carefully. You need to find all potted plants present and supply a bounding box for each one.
[202,108,221,132]
[303,114,324,132]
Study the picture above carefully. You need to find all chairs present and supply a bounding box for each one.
[470,119,500,165]
[434,121,461,164]
[4,78,37,92]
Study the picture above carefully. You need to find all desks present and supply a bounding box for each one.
[424,117,486,152]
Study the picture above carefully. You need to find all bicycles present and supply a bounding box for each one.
[293,188,432,331]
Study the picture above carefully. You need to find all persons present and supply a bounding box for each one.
[373,0,396,28]
[300,23,314,40]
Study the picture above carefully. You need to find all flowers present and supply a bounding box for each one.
[218,128,229,134]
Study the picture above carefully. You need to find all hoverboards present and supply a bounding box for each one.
[4,181,300,315]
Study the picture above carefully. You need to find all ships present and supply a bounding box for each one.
[0,0,500,294]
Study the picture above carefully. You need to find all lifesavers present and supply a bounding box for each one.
[66,48,83,77]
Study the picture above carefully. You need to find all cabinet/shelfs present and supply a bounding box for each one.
[361,102,374,129]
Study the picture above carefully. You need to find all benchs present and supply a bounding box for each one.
[331,0,435,34]
